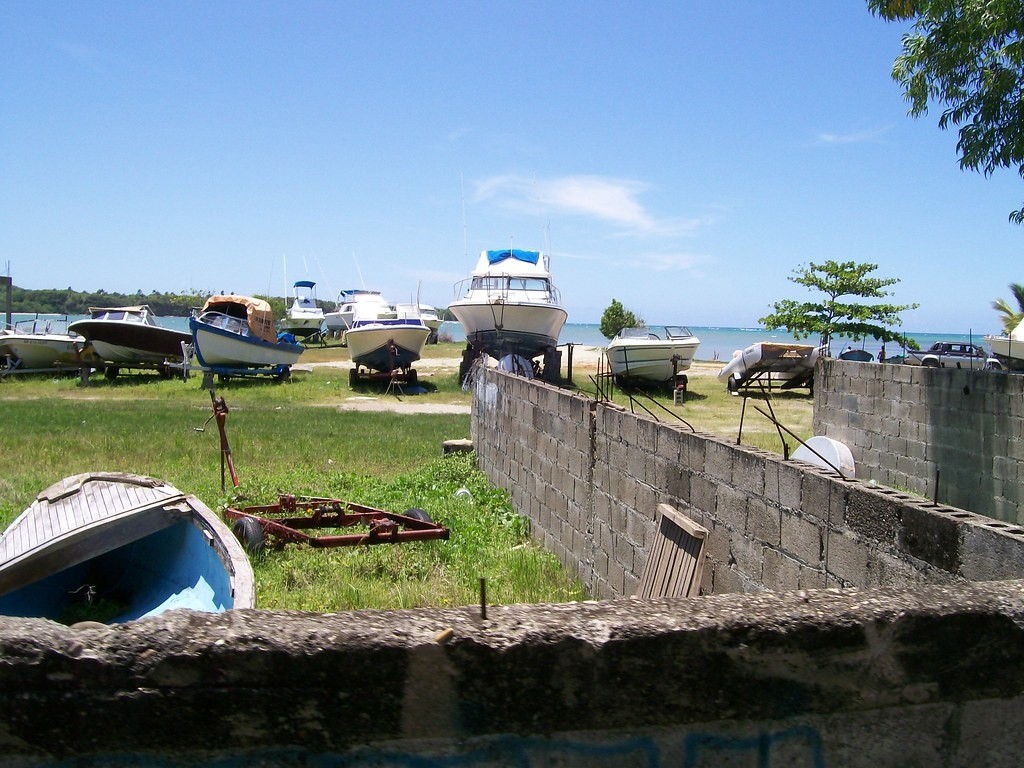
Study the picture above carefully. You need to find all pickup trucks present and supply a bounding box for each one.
[904,341,1003,371]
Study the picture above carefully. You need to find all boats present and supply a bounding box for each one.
[10,311,104,369]
[284,254,325,330]
[0,472,256,629]
[189,264,306,366]
[838,336,874,363]
[606,326,701,382]
[448,169,569,361]
[324,251,445,373]
[717,341,829,385]
[68,304,196,363]
